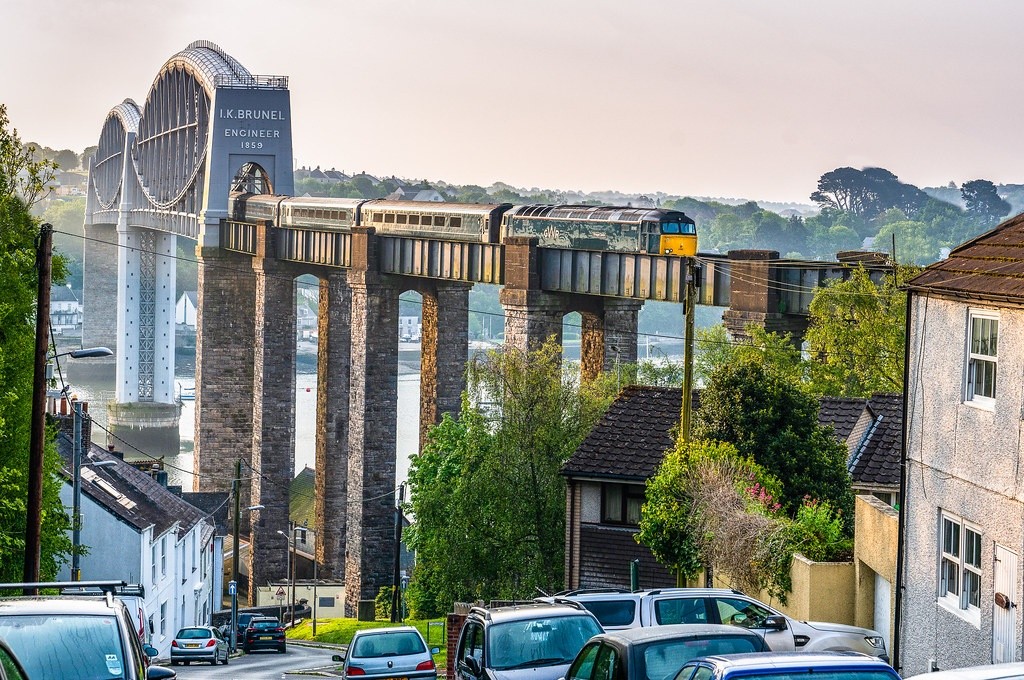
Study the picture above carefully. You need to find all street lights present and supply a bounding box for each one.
[24,346,114,596]
[231,505,266,654]
[71,460,118,582]
[277,530,290,612]
[292,520,308,628]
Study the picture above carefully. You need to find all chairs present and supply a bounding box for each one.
[694,600,706,623]
[360,641,382,656]
[679,606,690,624]
[397,637,417,654]
[495,634,517,665]
[543,630,574,658]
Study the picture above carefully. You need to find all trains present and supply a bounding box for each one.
[228,189,697,257]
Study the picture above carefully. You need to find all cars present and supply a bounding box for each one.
[170,625,230,666]
[663,650,903,680]
[332,626,440,680]
[564,624,772,680]
[0,580,176,680]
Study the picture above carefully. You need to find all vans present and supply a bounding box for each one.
[59,582,154,667]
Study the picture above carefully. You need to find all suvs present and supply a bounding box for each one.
[453,598,614,680]
[237,612,264,643]
[243,616,287,654]
[535,586,890,665]
